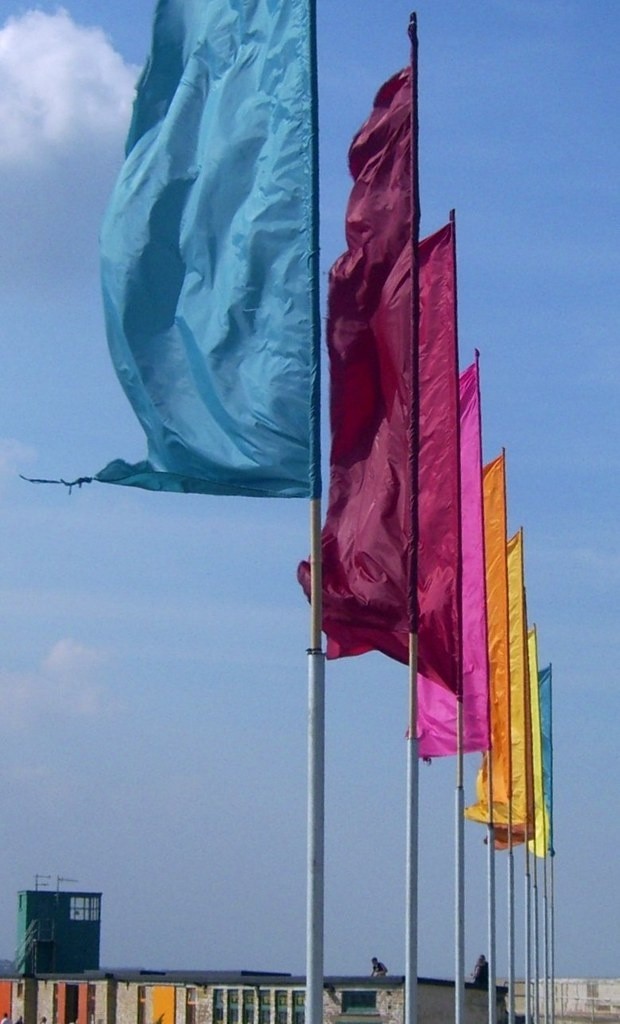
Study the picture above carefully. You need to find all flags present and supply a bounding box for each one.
[20,0,556,862]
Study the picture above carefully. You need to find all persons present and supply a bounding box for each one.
[472,954,488,985]
[1,1013,47,1024]
[370,957,388,976]
